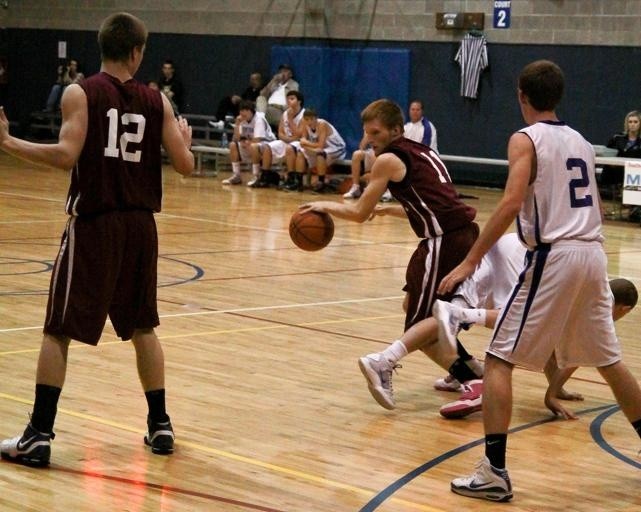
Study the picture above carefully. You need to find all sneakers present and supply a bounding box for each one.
[432,299,469,359]
[246,175,325,195]
[1,431,55,467]
[381,192,393,201]
[450,450,513,501]
[358,352,402,409]
[222,175,243,185]
[144,412,175,453]
[342,187,361,199]
[439,374,484,418]
[434,356,484,391]
[209,120,225,129]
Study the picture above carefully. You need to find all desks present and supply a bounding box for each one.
[594,155,641,221]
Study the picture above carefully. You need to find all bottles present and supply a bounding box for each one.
[222,132,228,149]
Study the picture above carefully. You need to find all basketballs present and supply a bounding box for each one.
[289,207,334,250]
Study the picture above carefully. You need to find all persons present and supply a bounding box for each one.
[0,11,196,471]
[298,96,483,421]
[208,63,347,193]
[342,125,385,200]
[595,110,640,198]
[358,231,638,420]
[146,63,188,158]
[379,100,439,203]
[433,60,641,504]
[48,58,86,109]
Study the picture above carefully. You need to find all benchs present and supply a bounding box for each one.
[438,152,606,216]
